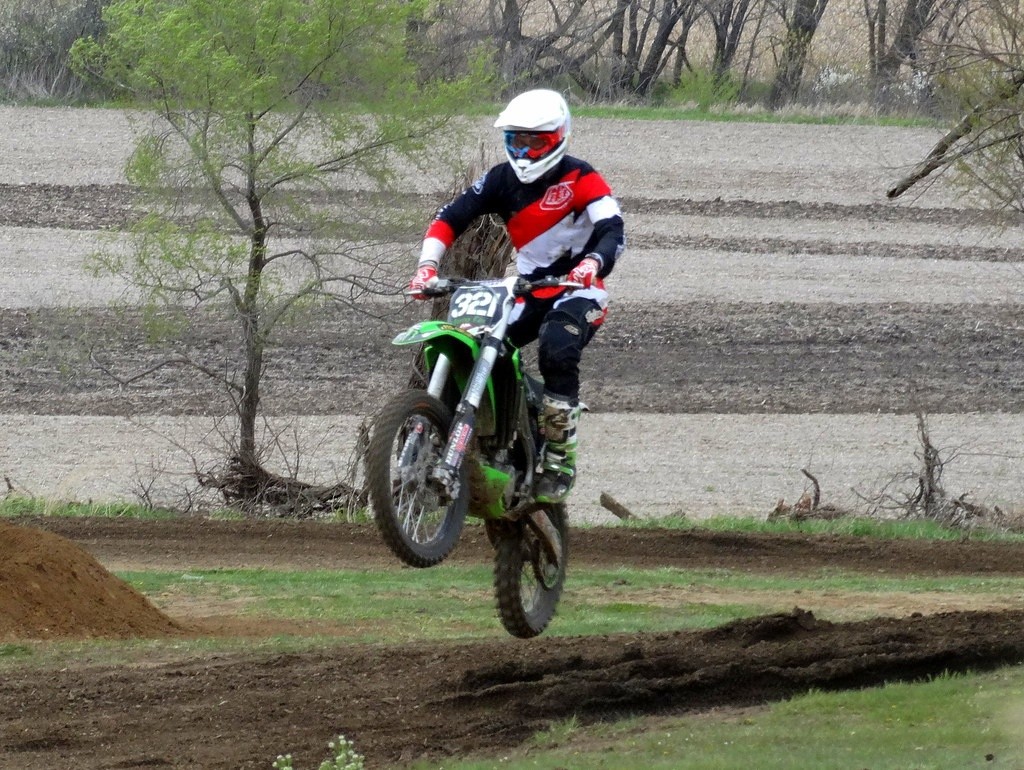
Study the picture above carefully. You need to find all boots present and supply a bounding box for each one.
[528,390,590,502]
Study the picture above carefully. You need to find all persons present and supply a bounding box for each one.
[412,90,624,502]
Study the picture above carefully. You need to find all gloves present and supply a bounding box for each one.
[565,261,598,295]
[409,265,440,301]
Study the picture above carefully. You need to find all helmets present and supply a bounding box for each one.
[492,89,571,183]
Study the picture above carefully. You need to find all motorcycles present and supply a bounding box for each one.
[364,271,591,640]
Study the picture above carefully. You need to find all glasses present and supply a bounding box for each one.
[504,131,546,150]
